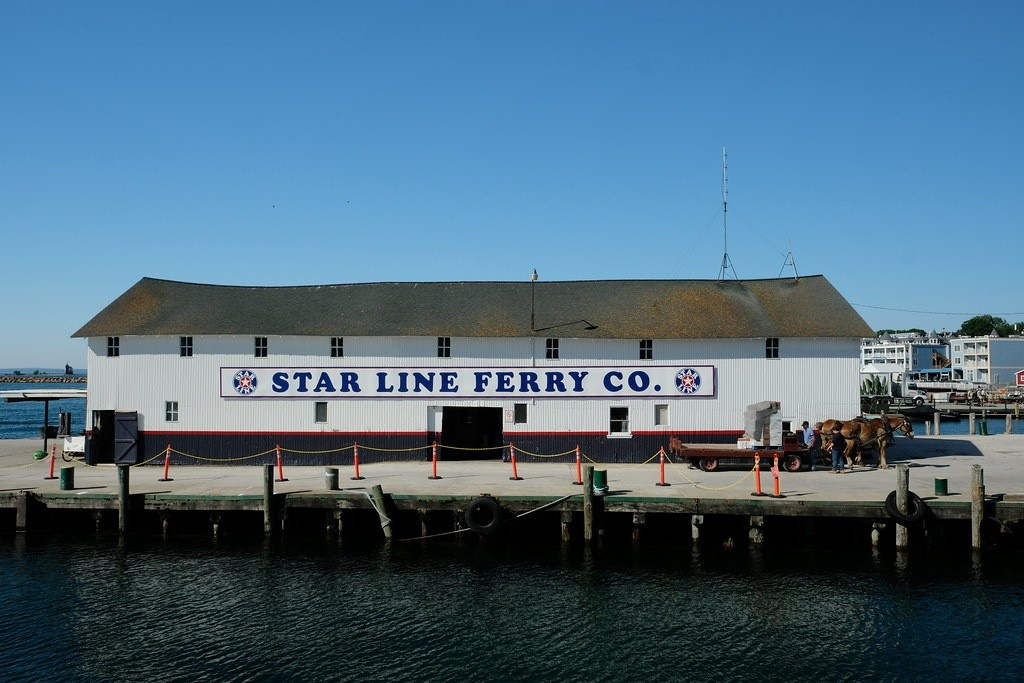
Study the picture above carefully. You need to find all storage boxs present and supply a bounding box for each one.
[736,440,751,449]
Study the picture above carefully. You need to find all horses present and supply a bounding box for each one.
[815,417,895,467]
[840,417,915,470]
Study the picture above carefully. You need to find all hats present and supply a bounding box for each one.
[800,421,809,426]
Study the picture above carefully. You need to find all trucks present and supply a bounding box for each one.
[890,390,929,407]
[666,431,813,472]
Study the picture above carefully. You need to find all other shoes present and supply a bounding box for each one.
[805,467,814,471]
[839,471,844,474]
[828,470,836,474]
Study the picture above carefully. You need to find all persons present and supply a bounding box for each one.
[801,421,817,471]
[967,385,984,406]
[827,426,845,474]
